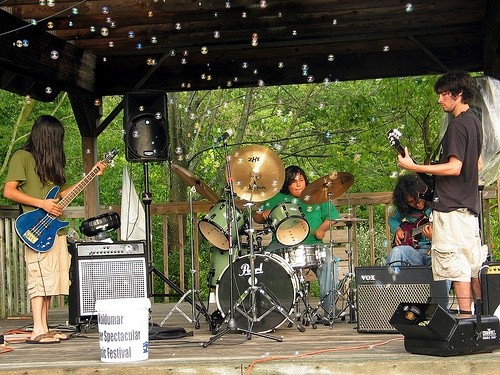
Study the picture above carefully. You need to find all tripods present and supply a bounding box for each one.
[141,143,358,347]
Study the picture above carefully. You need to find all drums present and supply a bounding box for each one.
[270,244,318,269]
[235,218,273,247]
[197,198,248,251]
[268,201,311,247]
[310,243,330,268]
[209,244,247,286]
[215,251,300,334]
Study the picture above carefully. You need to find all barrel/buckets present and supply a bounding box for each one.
[95,297,153,363]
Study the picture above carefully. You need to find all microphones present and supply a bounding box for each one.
[218,128,235,141]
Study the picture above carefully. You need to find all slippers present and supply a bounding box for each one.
[26,330,70,344]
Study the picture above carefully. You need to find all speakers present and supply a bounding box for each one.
[480,263,500,315]
[123,91,169,162]
[354,267,448,333]
[66,241,149,324]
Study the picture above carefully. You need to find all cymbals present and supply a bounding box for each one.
[171,162,219,203]
[225,144,286,204]
[332,218,370,222]
[300,171,355,205]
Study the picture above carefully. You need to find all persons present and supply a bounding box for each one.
[386,175,433,269]
[254,165,344,320]
[3,115,108,344]
[396,69,483,320]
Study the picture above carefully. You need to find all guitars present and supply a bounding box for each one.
[14,147,118,253]
[386,128,439,201]
[391,213,433,250]
